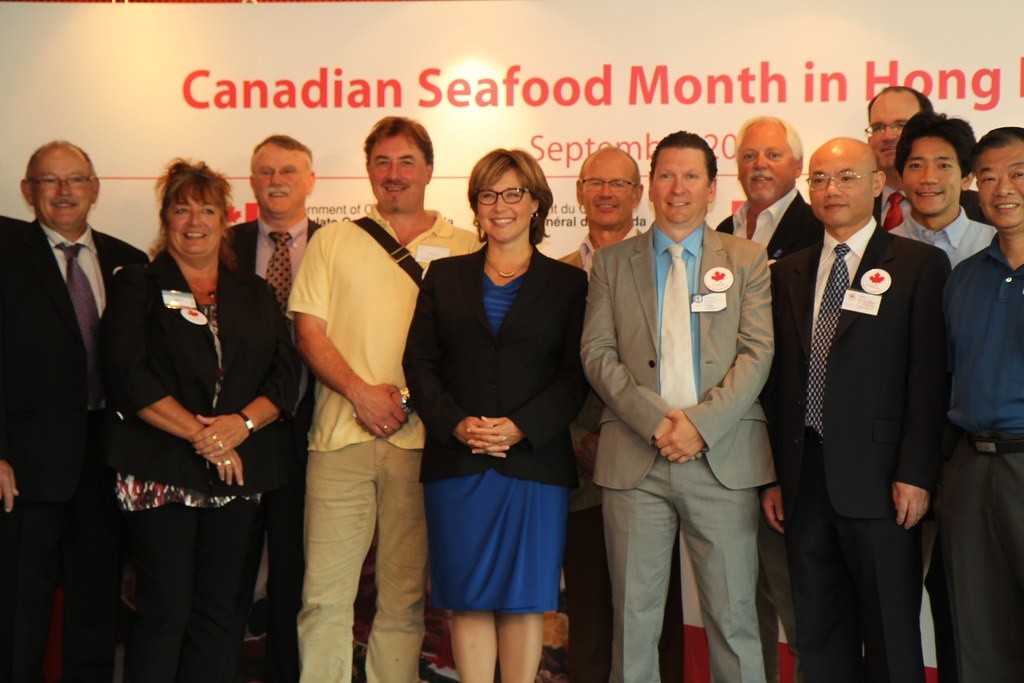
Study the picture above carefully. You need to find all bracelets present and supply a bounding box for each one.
[235,411,254,437]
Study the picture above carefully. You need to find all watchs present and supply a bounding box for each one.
[399,387,416,414]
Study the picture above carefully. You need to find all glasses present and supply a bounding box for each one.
[805,170,879,190]
[474,187,530,206]
[580,178,638,193]
[26,175,95,190]
[865,120,908,137]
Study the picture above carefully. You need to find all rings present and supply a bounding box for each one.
[218,441,224,449]
[224,460,231,465]
[216,462,224,466]
[212,433,219,442]
[383,425,389,431]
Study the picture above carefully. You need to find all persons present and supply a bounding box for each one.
[96,163,309,682]
[581,131,777,683]
[401,148,590,683]
[924,125,1024,683]
[867,86,997,233]
[1,140,150,682]
[288,117,488,682]
[560,147,684,683]
[887,112,1001,270]
[224,135,322,682]
[760,136,953,682]
[715,115,825,262]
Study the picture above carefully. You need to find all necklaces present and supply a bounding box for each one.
[190,284,217,299]
[486,245,533,277]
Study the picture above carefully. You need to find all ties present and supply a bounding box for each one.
[659,243,698,410]
[805,243,850,439]
[882,191,905,232]
[54,241,105,410]
[264,231,292,336]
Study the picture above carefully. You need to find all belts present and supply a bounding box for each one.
[958,431,1024,456]
